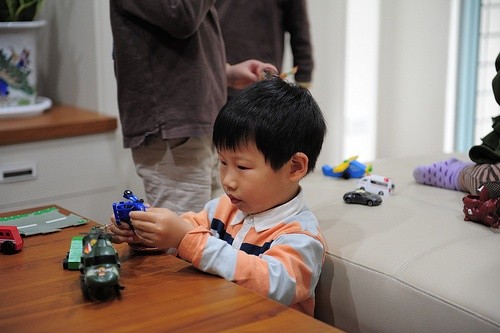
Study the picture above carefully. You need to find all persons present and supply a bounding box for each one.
[109,77,326,317]
[110,0,278,216]
[413,157,500,196]
[214,0,314,101]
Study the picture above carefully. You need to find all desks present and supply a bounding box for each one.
[0,204,349,333]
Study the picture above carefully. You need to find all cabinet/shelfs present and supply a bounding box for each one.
[0,132,124,226]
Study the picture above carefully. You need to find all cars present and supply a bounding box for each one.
[343,189,382,206]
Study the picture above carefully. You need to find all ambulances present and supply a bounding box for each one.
[358,174,396,197]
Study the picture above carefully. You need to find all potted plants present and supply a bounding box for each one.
[0,1,47,106]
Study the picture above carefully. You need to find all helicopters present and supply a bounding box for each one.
[77,223,126,302]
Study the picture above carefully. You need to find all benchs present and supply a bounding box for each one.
[210,152,500,333]
